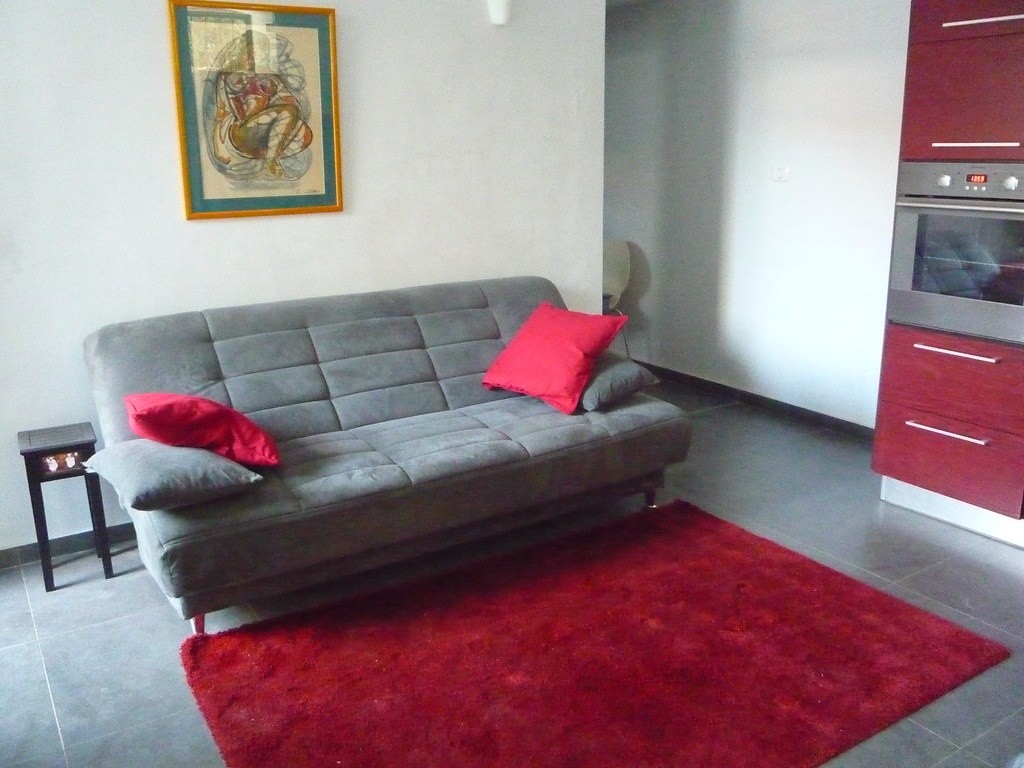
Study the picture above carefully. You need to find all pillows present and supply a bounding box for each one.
[578,350,663,413]
[80,439,263,510]
[125,392,280,468]
[482,302,629,415]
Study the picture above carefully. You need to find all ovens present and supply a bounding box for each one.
[888,161,1024,345]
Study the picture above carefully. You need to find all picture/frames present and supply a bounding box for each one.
[168,0,343,220]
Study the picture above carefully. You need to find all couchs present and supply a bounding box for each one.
[85,277,693,638]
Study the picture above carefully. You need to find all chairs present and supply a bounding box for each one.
[601,238,630,361]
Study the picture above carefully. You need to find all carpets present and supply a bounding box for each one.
[178,497,1009,768]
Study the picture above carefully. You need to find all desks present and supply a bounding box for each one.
[17,422,113,593]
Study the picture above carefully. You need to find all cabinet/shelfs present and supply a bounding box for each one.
[871,0,1024,551]
